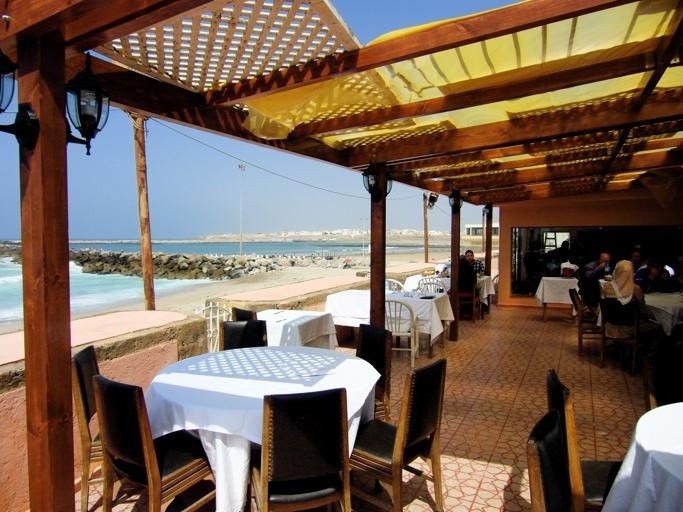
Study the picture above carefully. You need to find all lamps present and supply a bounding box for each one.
[232,101,250,113]
[67,52,116,155]
[362,146,395,201]
[0,43,39,151]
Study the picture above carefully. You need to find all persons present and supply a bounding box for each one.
[550,241,570,266]
[465,250,484,276]
[458,255,477,320]
[600,260,663,369]
[582,249,683,312]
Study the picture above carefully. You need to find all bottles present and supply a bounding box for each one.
[448,260,452,277]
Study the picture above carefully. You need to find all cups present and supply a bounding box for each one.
[605,263,610,271]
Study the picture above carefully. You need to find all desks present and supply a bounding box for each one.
[601,401,683,512]
[325,290,455,358]
[596,291,683,336]
[402,273,496,321]
[257,310,339,347]
[143,346,381,512]
[534,275,580,319]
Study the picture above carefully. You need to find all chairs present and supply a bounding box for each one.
[547,368,623,512]
[385,277,447,293]
[219,320,267,351]
[71,345,104,512]
[356,324,393,421]
[526,409,572,512]
[93,372,217,511]
[248,388,353,512]
[385,299,419,368]
[232,307,257,322]
[570,289,660,375]
[348,356,447,512]
